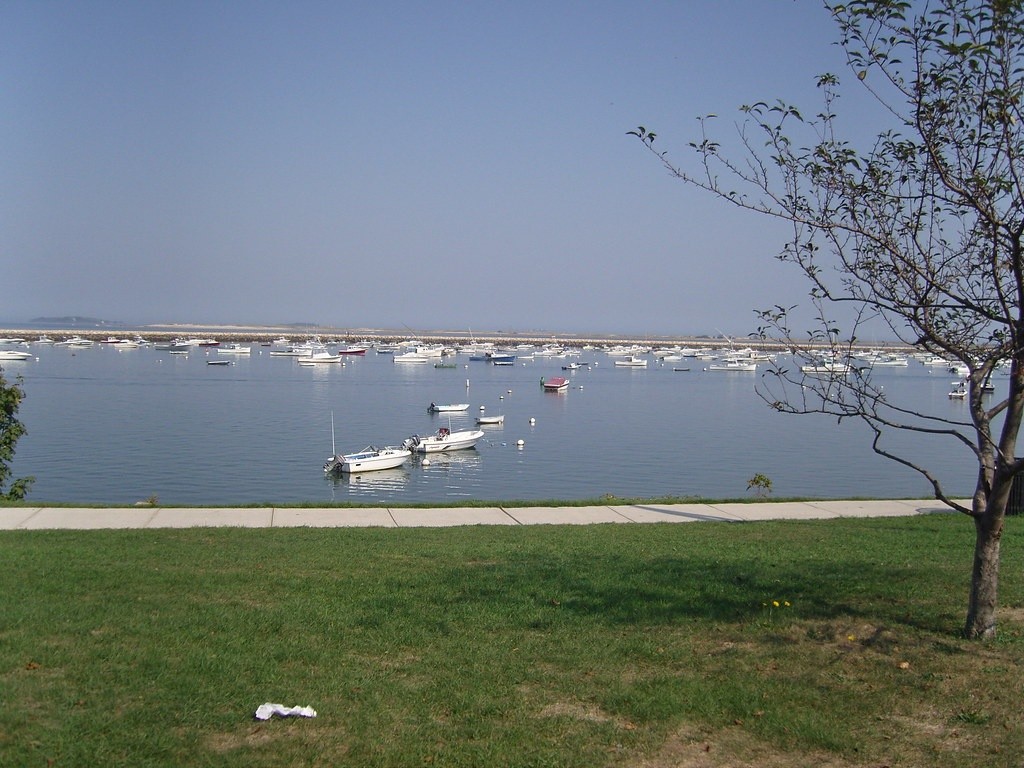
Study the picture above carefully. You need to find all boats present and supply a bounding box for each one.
[981,380,996,394]
[948,380,967,400]
[544,376,570,394]
[474,414,506,424]
[400,416,486,452]
[0,336,1015,373]
[432,403,470,411]
[322,412,412,474]
